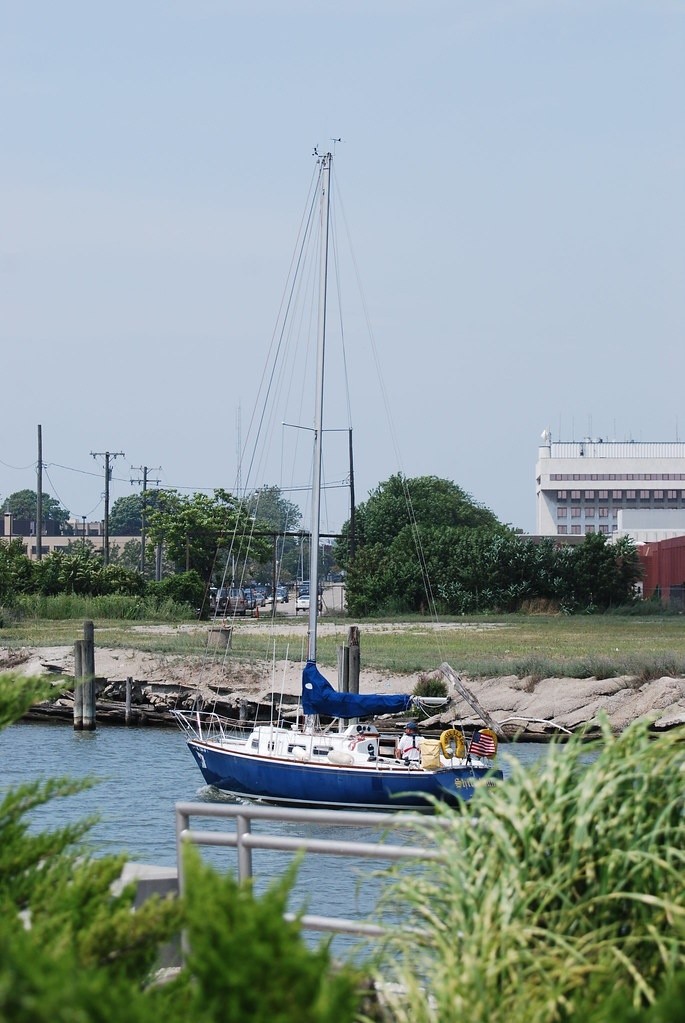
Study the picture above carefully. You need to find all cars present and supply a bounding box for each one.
[296,581,323,611]
[333,575,344,583]
[244,584,294,610]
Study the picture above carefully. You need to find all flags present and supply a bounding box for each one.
[469,730,495,758]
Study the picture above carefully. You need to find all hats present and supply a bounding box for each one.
[403,722,419,731]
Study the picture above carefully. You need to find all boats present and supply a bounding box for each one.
[168,137,502,812]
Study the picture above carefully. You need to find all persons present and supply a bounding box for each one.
[396,722,425,762]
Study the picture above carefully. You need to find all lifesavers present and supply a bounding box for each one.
[440,728,466,760]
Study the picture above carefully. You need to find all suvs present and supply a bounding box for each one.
[209,587,246,616]
